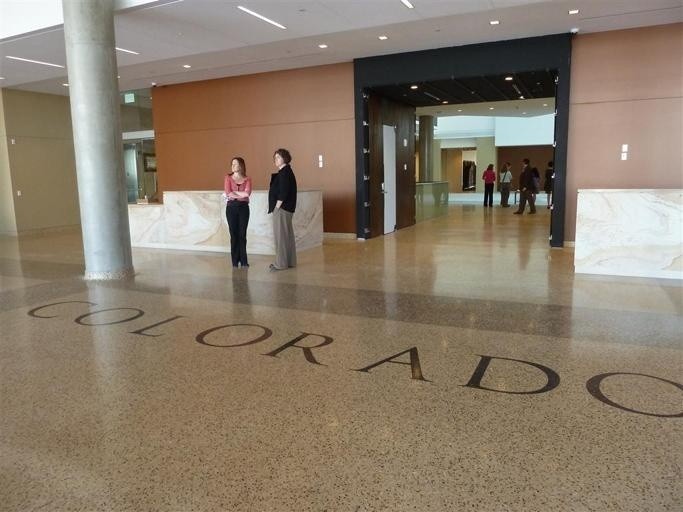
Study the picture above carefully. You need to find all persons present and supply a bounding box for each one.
[544,161,553,210]
[512,158,537,215]
[528,167,542,206]
[499,161,513,208]
[482,164,496,207]
[224,156,253,267]
[267,148,298,271]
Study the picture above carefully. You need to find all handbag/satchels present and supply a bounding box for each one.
[496,182,503,192]
